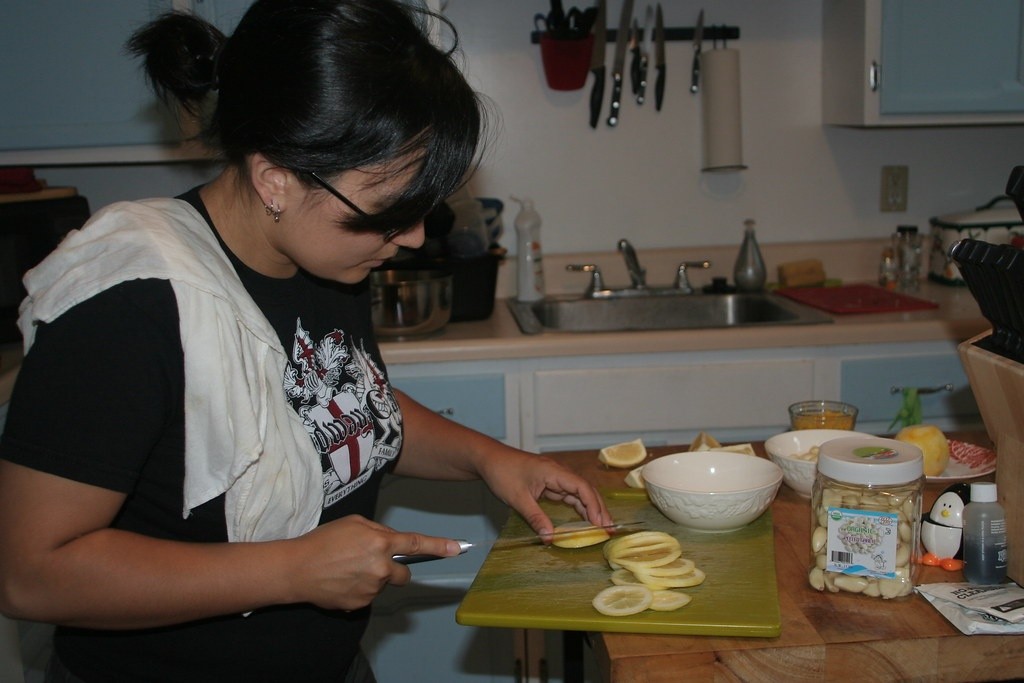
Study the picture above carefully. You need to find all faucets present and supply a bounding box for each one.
[617,237,650,289]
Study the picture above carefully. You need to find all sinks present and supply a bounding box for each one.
[529,290,803,329]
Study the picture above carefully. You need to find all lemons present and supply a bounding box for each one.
[625,430,755,489]
[550,521,705,617]
[598,438,647,467]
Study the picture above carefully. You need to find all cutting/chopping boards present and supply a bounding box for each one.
[455,488,783,637]
[772,284,939,314]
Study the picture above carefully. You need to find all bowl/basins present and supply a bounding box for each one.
[765,428,874,500]
[640,450,784,534]
[370,270,453,340]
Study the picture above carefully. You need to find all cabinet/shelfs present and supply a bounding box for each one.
[822,0,1024,126]
[364,343,969,683]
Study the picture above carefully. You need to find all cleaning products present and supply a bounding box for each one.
[510,191,546,305]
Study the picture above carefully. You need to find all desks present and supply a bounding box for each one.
[542,442,1024,683]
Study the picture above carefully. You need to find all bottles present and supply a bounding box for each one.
[808,438,927,604]
[894,225,920,293]
[733,218,766,290]
[877,248,899,290]
[962,482,1008,587]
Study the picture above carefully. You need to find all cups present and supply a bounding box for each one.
[539,31,595,91]
[788,400,858,431]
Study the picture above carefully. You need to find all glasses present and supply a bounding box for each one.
[310,171,429,243]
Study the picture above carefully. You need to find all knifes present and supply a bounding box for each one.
[392,521,646,566]
[587,0,706,128]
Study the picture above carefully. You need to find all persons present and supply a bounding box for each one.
[0,0,615,683]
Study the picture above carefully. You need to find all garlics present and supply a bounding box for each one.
[787,447,819,463]
[810,484,918,598]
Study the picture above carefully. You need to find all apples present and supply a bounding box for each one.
[895,424,950,477]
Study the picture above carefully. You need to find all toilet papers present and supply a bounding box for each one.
[698,48,749,174]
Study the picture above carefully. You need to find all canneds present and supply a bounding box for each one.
[808,436,924,599]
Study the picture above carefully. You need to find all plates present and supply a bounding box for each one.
[923,439,998,483]
